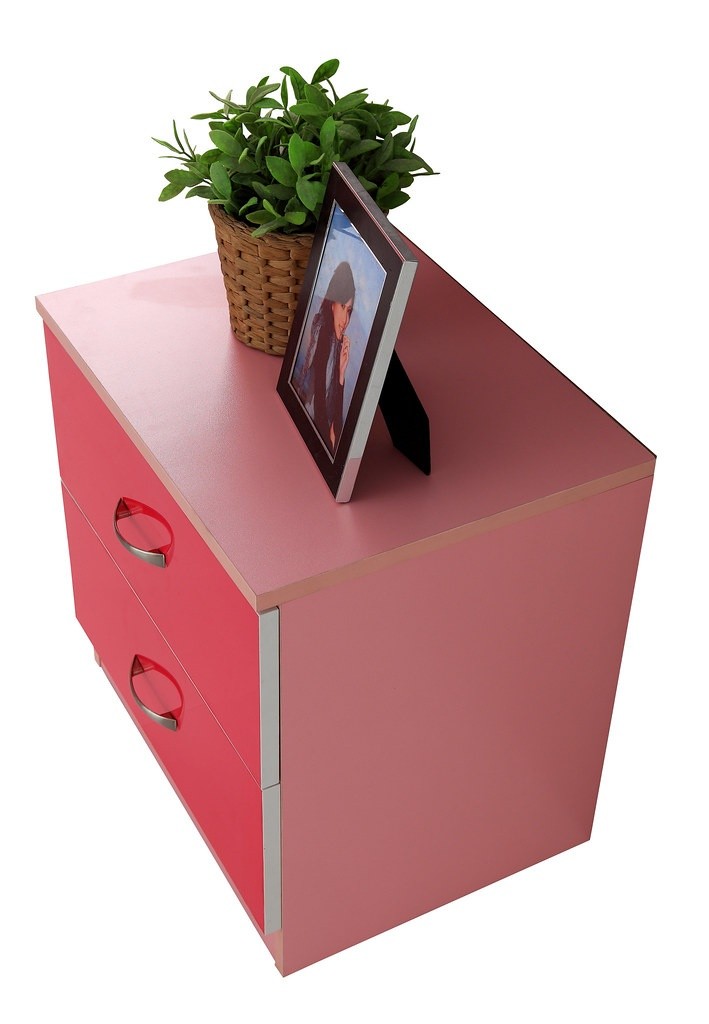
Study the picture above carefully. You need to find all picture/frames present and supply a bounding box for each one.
[276,162,431,504]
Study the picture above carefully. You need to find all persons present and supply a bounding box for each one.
[298,261,356,459]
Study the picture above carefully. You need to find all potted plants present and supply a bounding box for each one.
[151,58,441,358]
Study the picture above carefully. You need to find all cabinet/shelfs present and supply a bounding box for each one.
[34,228,659,978]
